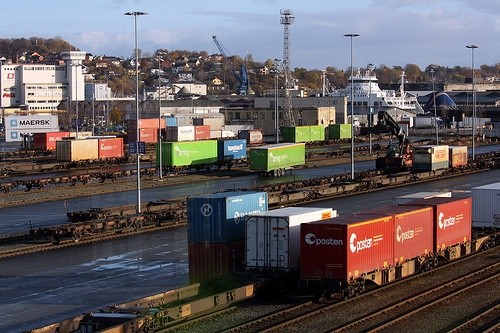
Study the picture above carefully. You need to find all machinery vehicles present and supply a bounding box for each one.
[212,35,248,95]
[376,109,414,172]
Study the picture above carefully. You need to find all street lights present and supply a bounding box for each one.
[125,12,149,213]
[343,33,361,181]
[465,44,479,160]
